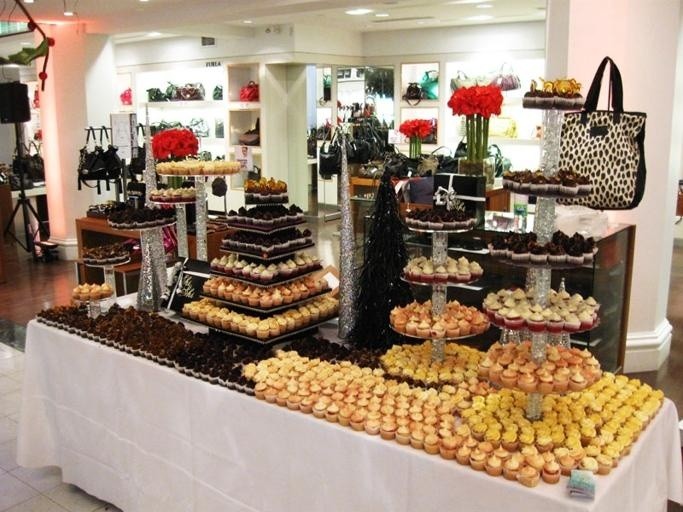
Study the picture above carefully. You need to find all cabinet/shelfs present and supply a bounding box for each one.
[399,97,439,153]
[228,101,261,191]
[76,217,176,295]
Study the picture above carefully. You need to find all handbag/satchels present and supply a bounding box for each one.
[308,68,521,180]
[9,140,45,190]
[556,56,647,210]
[76,125,122,195]
[120,79,261,173]
[247,165,261,182]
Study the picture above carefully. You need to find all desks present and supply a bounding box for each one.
[13,294,681,512]
[347,170,511,239]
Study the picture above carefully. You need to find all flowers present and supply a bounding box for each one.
[398,120,434,160]
[446,83,504,160]
[152,128,199,190]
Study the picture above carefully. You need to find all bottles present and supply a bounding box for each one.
[515,191,529,224]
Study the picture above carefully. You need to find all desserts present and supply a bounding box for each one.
[36,159,665,486]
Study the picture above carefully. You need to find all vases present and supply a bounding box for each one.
[457,154,496,186]
